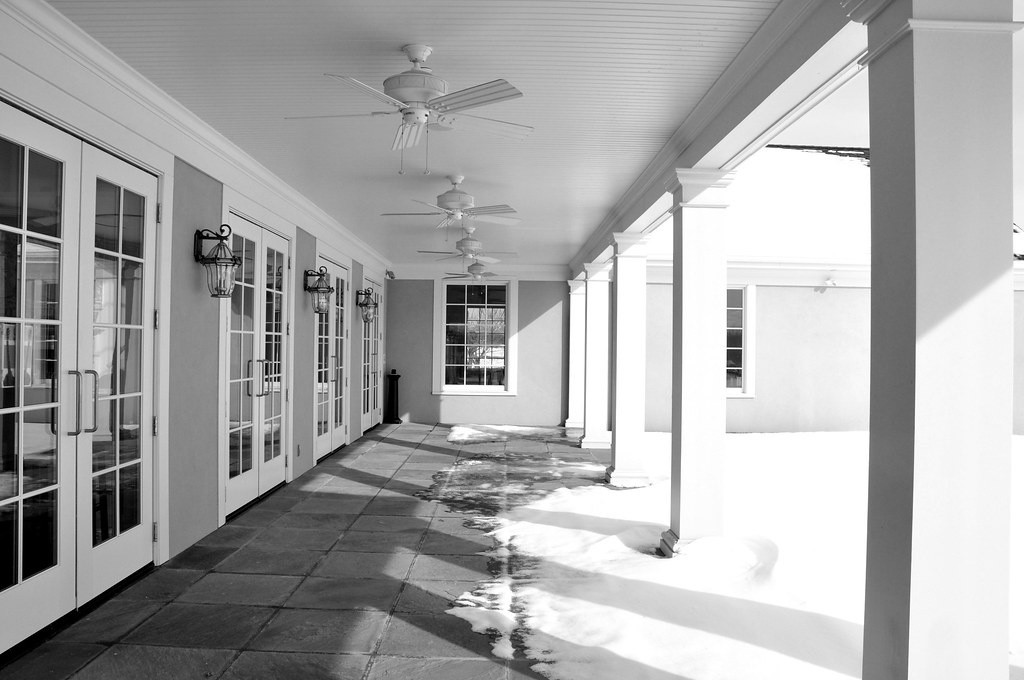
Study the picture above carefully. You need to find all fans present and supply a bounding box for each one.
[281,43,535,282]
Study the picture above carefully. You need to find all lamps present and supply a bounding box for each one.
[304,266,334,314]
[194,224,242,297]
[356,287,379,323]
